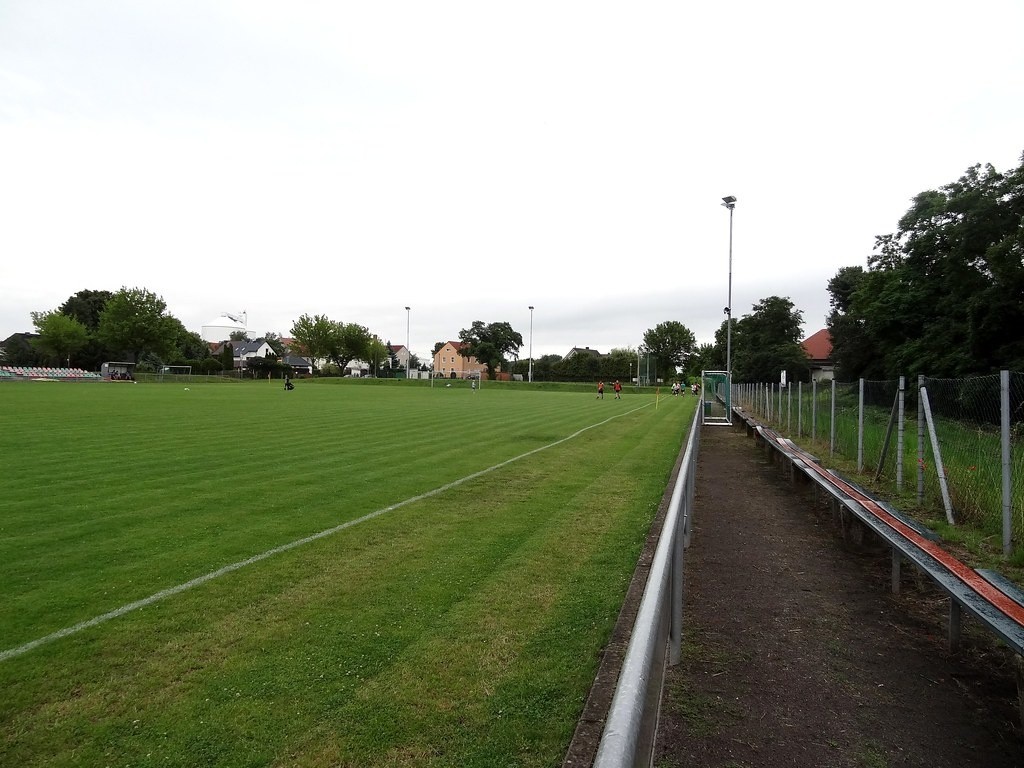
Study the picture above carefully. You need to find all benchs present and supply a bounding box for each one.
[716,392,1024,660]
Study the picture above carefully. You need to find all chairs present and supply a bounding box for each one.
[0,367,101,378]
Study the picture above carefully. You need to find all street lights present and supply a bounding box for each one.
[405,307,411,378]
[721,195,737,380]
[528,306,534,383]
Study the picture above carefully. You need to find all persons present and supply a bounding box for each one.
[284,375,289,390]
[112,370,131,380]
[596,380,622,399]
[472,380,477,392]
[671,381,700,397]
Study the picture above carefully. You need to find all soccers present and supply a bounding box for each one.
[134,381,137,384]
[596,395,600,399]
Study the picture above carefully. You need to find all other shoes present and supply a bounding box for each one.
[602,397,603,399]
[614,397,617,399]
[619,397,620,399]
